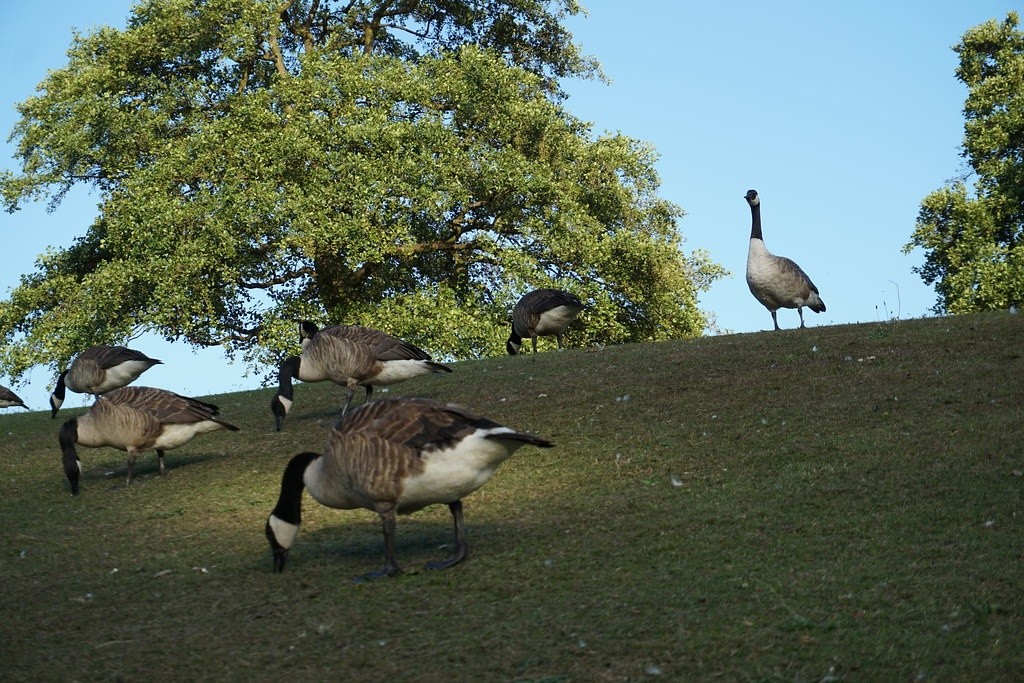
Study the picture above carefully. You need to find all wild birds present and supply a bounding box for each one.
[265,321,454,432]
[506,289,588,356]
[744,190,826,332]
[50,346,164,419]
[264,398,558,583]
[58,386,241,497]
[0,385,30,410]
